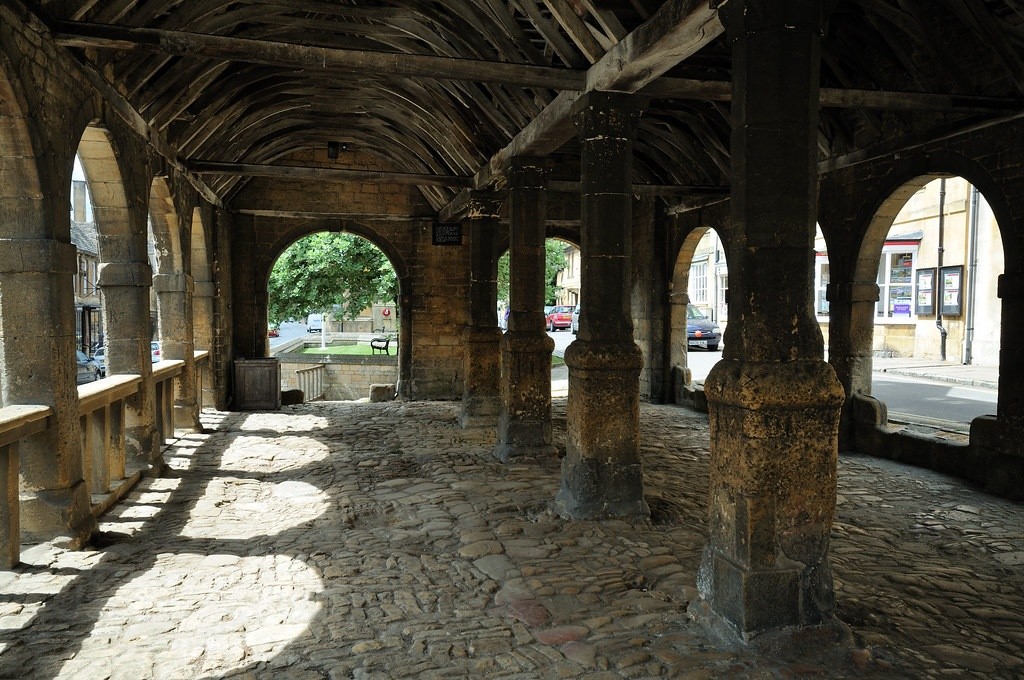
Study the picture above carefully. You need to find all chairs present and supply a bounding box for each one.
[372,325,385,333]
[372,334,393,355]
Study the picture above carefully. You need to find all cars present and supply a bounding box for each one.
[686,302,721,351]
[570,305,579,333]
[268,328,279,337]
[75,350,102,385]
[94,347,105,372]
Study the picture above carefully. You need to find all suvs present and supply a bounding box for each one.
[285,316,293,323]
[545,305,576,332]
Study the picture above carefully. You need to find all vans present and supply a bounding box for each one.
[306,314,322,333]
[151,341,161,363]
